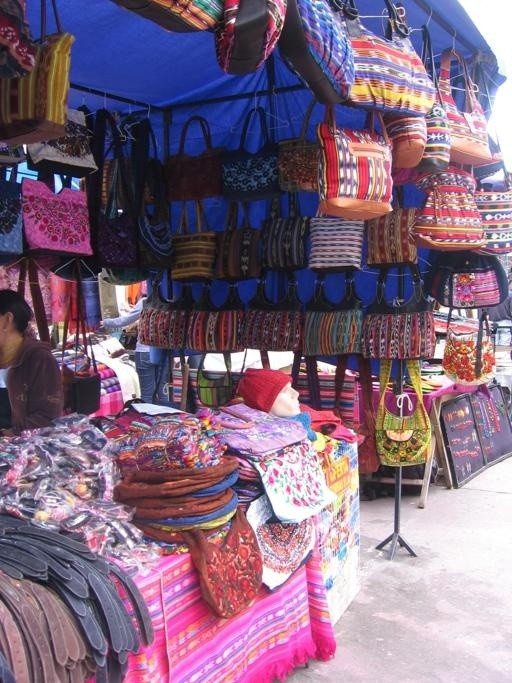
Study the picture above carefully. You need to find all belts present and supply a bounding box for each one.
[0,516,154,683]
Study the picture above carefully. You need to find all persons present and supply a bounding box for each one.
[98,294,172,404]
[236,368,301,418]
[0,287,63,437]
